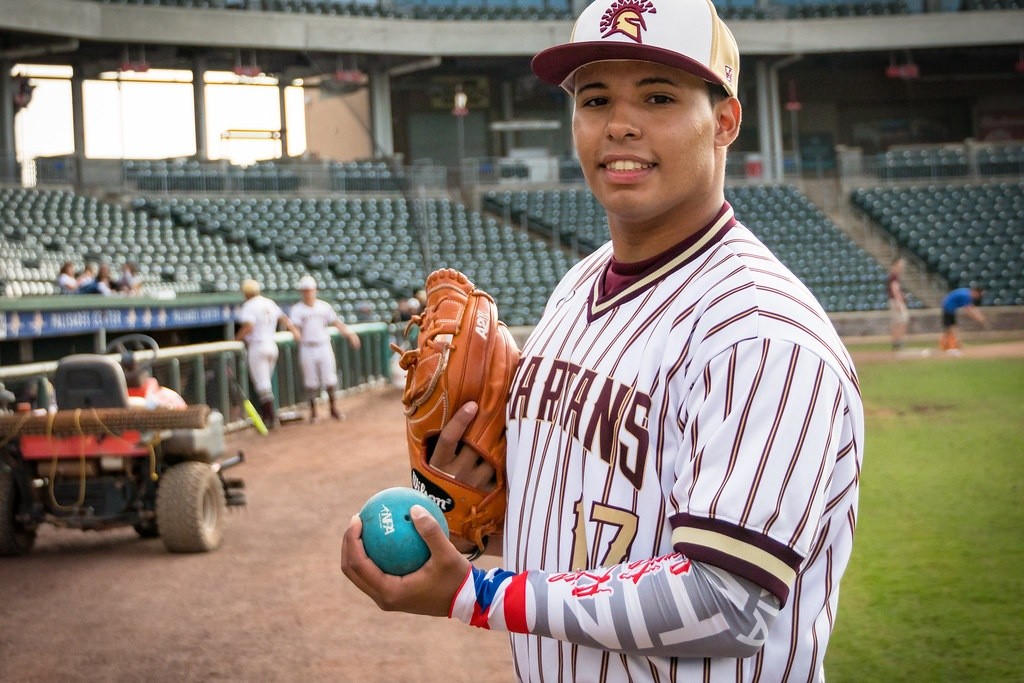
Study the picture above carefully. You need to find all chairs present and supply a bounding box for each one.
[0,142,1024,333]
[55,354,129,408]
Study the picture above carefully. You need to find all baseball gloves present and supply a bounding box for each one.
[383,266,524,551]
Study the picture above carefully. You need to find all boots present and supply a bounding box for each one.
[259,394,275,431]
[326,384,346,421]
[306,387,318,424]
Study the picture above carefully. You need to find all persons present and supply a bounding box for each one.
[289,276,360,423]
[940,286,984,350]
[392,287,427,324]
[235,278,300,429]
[59,261,139,296]
[341,0,865,683]
[887,258,910,347]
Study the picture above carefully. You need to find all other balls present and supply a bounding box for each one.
[357,483,451,577]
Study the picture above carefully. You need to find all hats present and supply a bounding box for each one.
[296,275,318,290]
[242,279,260,295]
[530,1,740,98]
